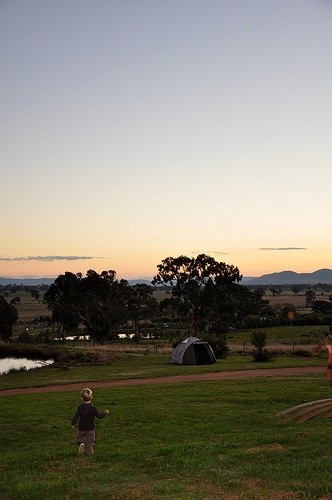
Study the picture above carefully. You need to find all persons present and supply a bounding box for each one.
[71,388,110,455]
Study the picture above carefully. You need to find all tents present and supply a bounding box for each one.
[170,336,217,364]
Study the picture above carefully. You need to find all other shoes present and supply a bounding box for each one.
[77,442,85,455]
